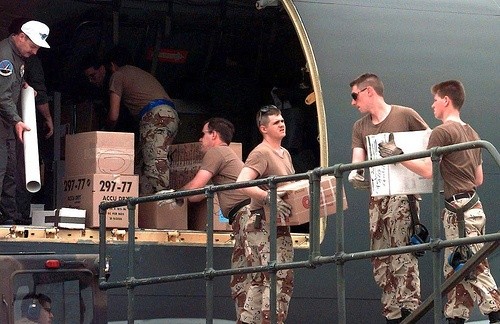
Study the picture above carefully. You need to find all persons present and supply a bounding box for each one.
[16,293,54,324]
[0,21,50,226]
[377,80,500,324]
[235,104,295,324]
[84,56,180,197]
[157,117,261,324]
[9,19,54,139]
[348,74,432,324]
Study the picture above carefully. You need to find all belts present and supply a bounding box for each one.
[136,99,175,122]
[447,192,474,203]
[250,207,264,217]
[228,197,251,225]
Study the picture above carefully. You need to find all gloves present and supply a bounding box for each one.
[379,133,404,166]
[156,189,184,211]
[347,169,371,190]
[264,191,293,220]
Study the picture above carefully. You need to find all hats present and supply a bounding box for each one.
[21,21,51,49]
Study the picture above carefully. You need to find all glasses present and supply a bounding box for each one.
[351,88,367,101]
[198,131,212,138]
[43,307,52,314]
[260,104,277,121]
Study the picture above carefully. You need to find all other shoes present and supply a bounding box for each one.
[0,214,33,225]
[487,310,500,324]
[385,319,402,324]
[446,316,464,324]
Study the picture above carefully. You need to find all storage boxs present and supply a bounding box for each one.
[60,173,139,230]
[139,191,189,229]
[193,193,234,232]
[263,175,349,228]
[168,142,242,191]
[365,129,432,197]
[64,131,135,175]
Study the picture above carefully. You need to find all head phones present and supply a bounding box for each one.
[28,291,40,320]
[448,244,474,279]
[408,222,428,254]
